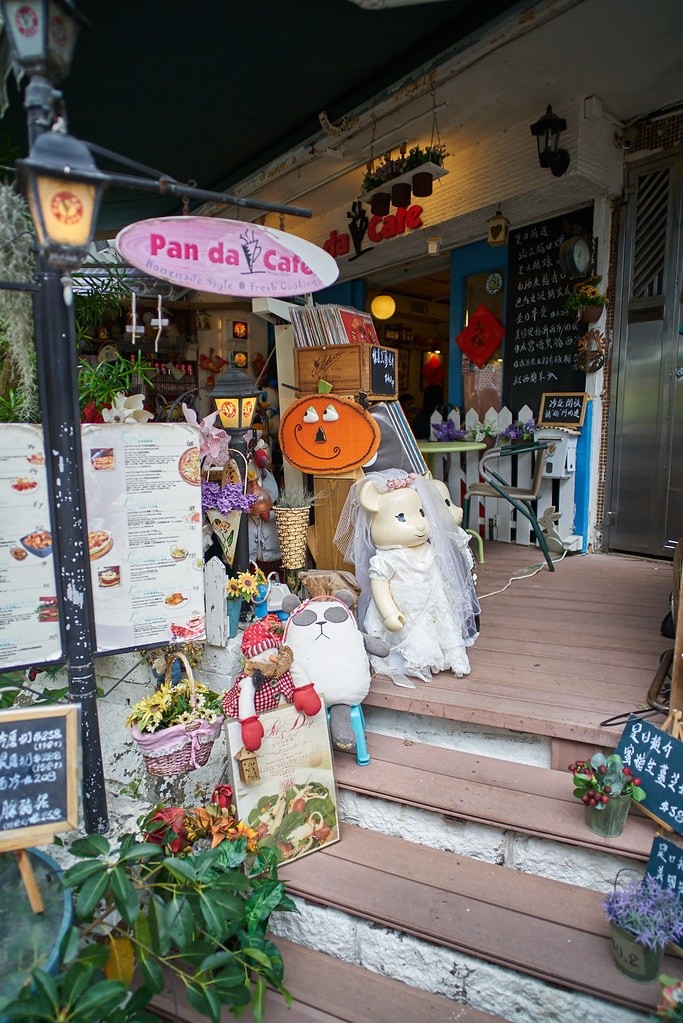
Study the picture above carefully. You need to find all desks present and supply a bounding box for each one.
[416,441,487,451]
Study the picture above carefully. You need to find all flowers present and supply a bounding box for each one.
[121,677,228,734]
[654,974,683,1023]
[603,871,683,951]
[199,480,262,515]
[432,418,539,442]
[144,784,260,856]
[226,569,260,603]
[360,143,449,192]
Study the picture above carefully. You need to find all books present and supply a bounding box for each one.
[78,354,195,391]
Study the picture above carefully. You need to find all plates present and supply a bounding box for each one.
[193,559,203,571]
[165,592,190,609]
[236,768,339,854]
[11,483,40,495]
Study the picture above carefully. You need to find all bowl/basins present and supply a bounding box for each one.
[89,531,111,555]
[170,550,188,561]
[178,447,200,486]
[19,529,53,558]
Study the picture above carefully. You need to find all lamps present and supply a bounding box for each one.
[211,367,262,432]
[371,295,396,320]
[530,104,570,178]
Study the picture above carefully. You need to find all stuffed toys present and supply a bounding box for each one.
[281,590,390,751]
[248,442,281,574]
[257,378,280,456]
[333,468,480,688]
[223,623,321,752]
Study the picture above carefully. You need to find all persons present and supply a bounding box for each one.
[399,394,416,425]
[412,384,447,440]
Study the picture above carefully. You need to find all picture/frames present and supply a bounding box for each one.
[420,351,446,391]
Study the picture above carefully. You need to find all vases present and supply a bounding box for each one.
[479,438,529,449]
[371,172,434,216]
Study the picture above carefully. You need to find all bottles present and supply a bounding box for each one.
[130,354,196,393]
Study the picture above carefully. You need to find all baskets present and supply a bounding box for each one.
[131,653,223,776]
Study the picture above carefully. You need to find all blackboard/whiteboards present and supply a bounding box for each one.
[502,200,595,424]
[360,341,398,400]
[538,392,588,427]
[640,832,683,923]
[612,712,683,837]
[0,702,83,854]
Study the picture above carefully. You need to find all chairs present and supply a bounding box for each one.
[461,441,560,571]
